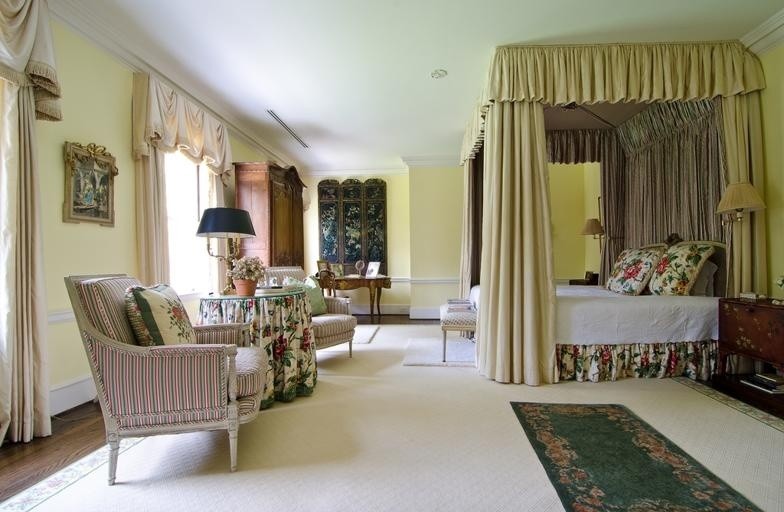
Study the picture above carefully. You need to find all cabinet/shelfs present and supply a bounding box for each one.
[230,161,307,272]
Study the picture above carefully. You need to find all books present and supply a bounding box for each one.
[255,285,304,295]
[739,372,784,394]
[446,299,476,313]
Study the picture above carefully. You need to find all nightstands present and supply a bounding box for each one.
[712,297,784,418]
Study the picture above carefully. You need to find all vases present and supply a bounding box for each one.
[232,280,257,295]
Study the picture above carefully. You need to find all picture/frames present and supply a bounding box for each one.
[63,140,118,227]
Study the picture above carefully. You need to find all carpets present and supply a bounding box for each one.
[509,401,763,512]
[0,324,784,512]
[348,325,380,344]
[402,338,477,367]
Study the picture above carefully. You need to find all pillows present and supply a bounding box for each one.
[282,276,328,317]
[125,282,198,345]
[604,243,668,295]
[649,243,715,295]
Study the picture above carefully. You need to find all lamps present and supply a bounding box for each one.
[580,218,606,240]
[196,207,256,295]
[717,182,765,227]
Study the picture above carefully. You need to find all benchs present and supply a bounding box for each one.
[440,301,477,362]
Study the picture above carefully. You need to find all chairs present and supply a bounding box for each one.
[266,266,357,357]
[63,272,269,486]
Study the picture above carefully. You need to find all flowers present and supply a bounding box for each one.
[226,255,267,282]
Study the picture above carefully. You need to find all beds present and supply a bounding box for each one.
[471,241,727,384]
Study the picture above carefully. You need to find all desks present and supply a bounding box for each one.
[317,276,392,323]
[196,288,317,410]
